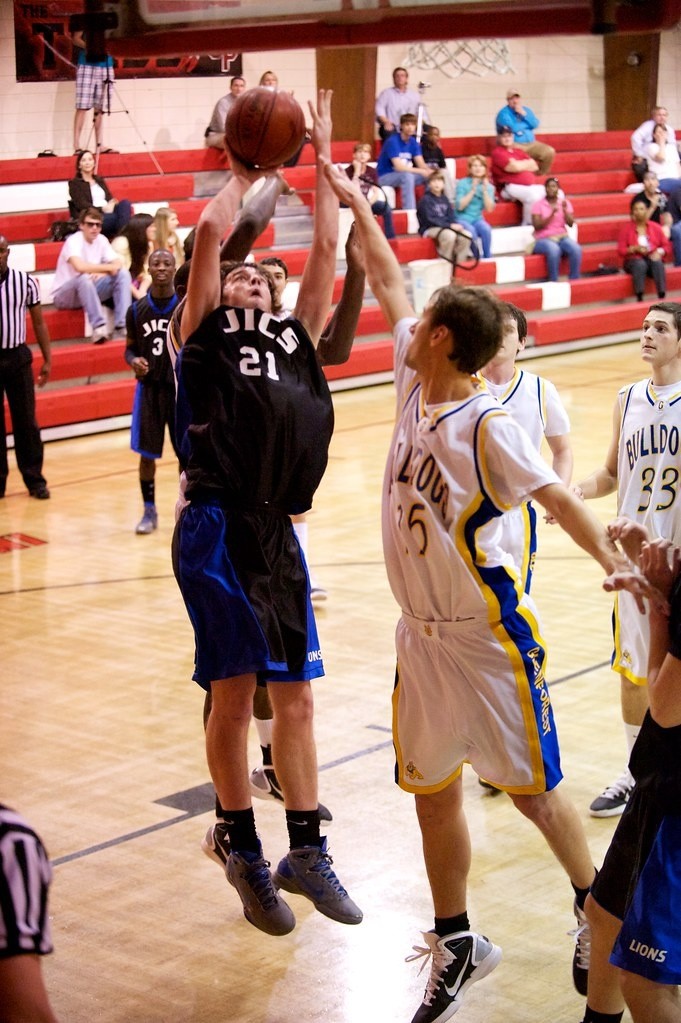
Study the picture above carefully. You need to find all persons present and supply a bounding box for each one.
[376,67,428,139]
[495,89,556,175]
[471,300,574,787]
[583,517,681,1023]
[420,127,457,203]
[72,31,120,154]
[124,250,177,536]
[454,153,496,258]
[530,177,581,281]
[165,90,367,936]
[568,302,681,817]
[317,156,670,1023]
[205,77,246,150]
[0,235,52,497]
[415,172,472,259]
[489,125,565,226]
[375,114,434,209]
[345,142,395,238]
[0,804,58,1023]
[51,206,185,345]
[259,71,278,87]
[68,150,131,238]
[618,106,681,302]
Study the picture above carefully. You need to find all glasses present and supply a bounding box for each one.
[82,222,100,228]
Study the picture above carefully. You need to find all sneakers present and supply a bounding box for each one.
[202,824,231,872]
[272,835,363,925]
[567,896,592,996]
[136,506,157,534]
[112,327,128,341]
[226,838,295,936]
[590,776,635,818]
[249,765,332,826]
[405,929,503,1023]
[92,325,108,344]
[29,486,49,499]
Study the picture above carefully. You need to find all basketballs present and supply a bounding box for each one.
[225,86,306,170]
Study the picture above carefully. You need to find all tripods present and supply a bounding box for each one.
[85,52,165,177]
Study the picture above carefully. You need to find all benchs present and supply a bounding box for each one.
[0,130,681,448]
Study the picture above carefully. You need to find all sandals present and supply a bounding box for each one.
[96,143,119,154]
[73,149,82,156]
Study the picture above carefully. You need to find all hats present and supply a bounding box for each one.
[507,89,520,100]
[498,126,512,134]
